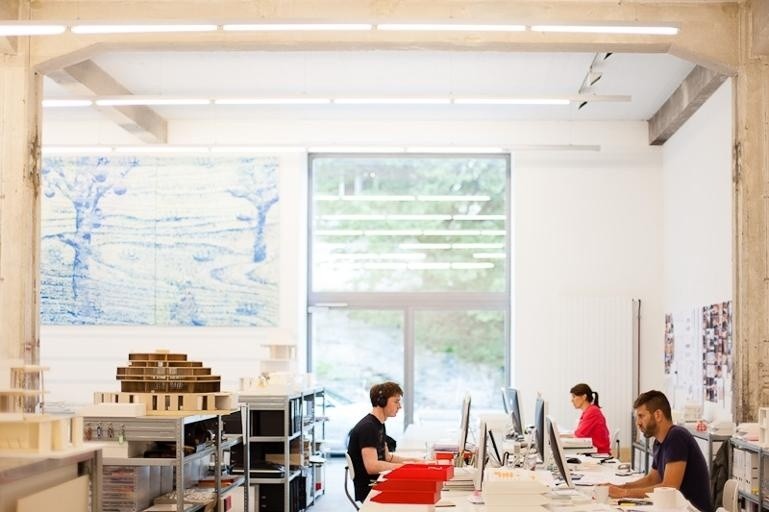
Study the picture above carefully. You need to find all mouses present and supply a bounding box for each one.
[567,458,581,463]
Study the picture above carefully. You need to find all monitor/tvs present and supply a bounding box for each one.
[505,388,523,445]
[540,416,582,499]
[523,397,547,472]
[468,416,509,504]
[459,390,477,471]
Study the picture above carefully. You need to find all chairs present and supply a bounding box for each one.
[343,450,362,512]
[612,428,621,459]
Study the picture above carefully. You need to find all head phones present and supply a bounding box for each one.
[377,384,387,407]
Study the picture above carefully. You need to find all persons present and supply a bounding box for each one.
[347,381,427,503]
[603,390,713,512]
[557,384,613,457]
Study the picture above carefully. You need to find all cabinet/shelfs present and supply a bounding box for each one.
[631,411,768,512]
[0,387,329,512]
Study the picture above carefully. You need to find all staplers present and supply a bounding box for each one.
[597,457,616,464]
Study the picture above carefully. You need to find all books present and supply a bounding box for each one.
[732,447,769,495]
[557,435,598,454]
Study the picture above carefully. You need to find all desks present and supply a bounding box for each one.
[359,424,700,512]
